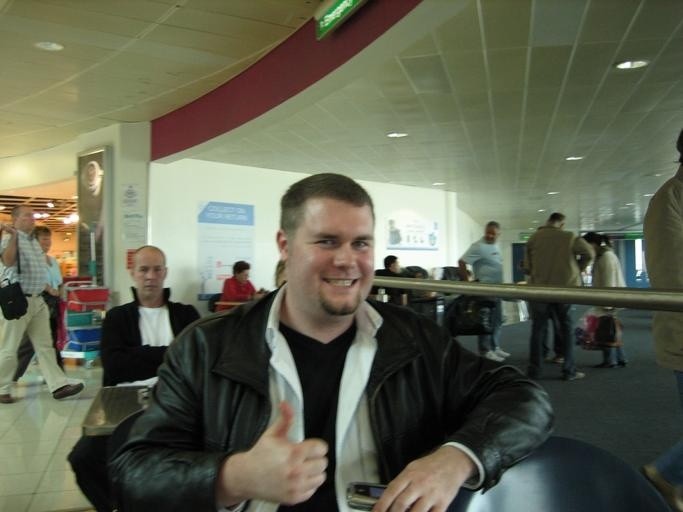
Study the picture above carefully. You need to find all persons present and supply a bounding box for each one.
[0,200,86,405]
[15,233,69,388]
[544,314,563,364]
[214,257,258,309]
[274,260,288,288]
[374,255,412,309]
[459,223,515,363]
[114,172,557,512]
[522,212,596,383]
[638,129,681,510]
[69,246,202,512]
[410,265,452,299]
[583,233,631,369]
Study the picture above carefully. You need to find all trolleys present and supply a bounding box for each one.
[55,278,112,368]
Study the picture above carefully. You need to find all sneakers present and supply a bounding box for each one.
[544,354,565,364]
[479,346,512,363]
[0,394,15,404]
[639,458,683,511]
[593,360,627,369]
[566,371,587,381]
[51,381,85,399]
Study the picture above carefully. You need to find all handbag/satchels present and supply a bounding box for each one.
[573,307,624,352]
[443,278,503,337]
[0,278,29,321]
[53,299,69,351]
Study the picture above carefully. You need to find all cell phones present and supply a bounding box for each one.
[346,482,387,511]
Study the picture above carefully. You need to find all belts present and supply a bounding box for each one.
[24,292,41,298]
